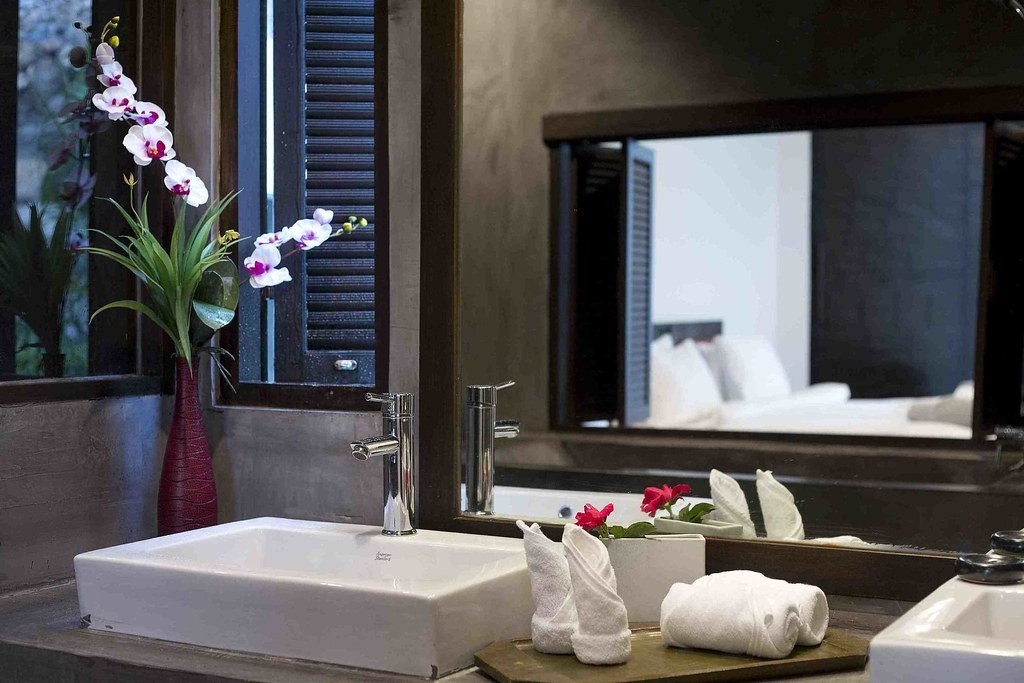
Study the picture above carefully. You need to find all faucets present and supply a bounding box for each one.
[350,391,414,535]
[464,376,522,517]
[957,527,1024,586]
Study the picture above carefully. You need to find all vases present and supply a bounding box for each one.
[654,516,744,540]
[157,356,219,537]
[598,534,706,624]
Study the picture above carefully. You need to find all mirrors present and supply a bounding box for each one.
[419,0,1024,602]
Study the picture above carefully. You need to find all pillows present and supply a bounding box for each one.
[650,339,725,425]
[712,335,795,403]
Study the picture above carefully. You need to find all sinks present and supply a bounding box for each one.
[462,482,714,537]
[869,574,1023,683]
[74,516,569,677]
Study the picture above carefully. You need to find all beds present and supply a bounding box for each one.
[646,321,974,440]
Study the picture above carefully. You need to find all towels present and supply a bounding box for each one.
[755,469,803,541]
[562,526,635,663]
[510,520,575,654]
[808,535,885,546]
[693,569,829,648]
[709,469,755,539]
[659,582,799,659]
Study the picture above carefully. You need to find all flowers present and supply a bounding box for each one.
[640,484,717,523]
[575,504,656,538]
[61,16,367,394]
